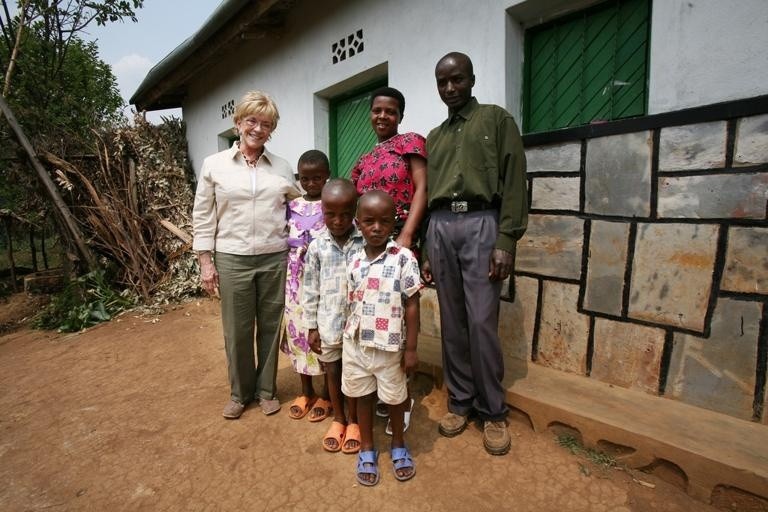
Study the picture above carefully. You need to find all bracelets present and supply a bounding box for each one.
[198,250,212,256]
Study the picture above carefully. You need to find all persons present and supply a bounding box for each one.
[348,88,431,437]
[193,91,302,421]
[280,149,333,424]
[417,53,531,455]
[327,190,423,490]
[302,179,367,456]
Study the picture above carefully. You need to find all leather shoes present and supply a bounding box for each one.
[439,410,473,439]
[481,419,513,457]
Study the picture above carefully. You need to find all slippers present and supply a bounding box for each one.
[376,398,390,417]
[384,397,415,436]
[307,397,333,423]
[389,442,416,483]
[288,392,319,420]
[341,422,362,454]
[321,416,348,452]
[357,446,381,487]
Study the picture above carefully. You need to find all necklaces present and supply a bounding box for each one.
[374,133,400,146]
[236,143,263,166]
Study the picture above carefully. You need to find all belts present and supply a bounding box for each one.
[432,200,501,213]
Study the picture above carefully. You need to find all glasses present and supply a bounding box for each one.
[242,119,274,130]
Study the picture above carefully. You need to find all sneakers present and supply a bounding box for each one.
[257,395,282,416]
[222,397,249,420]
